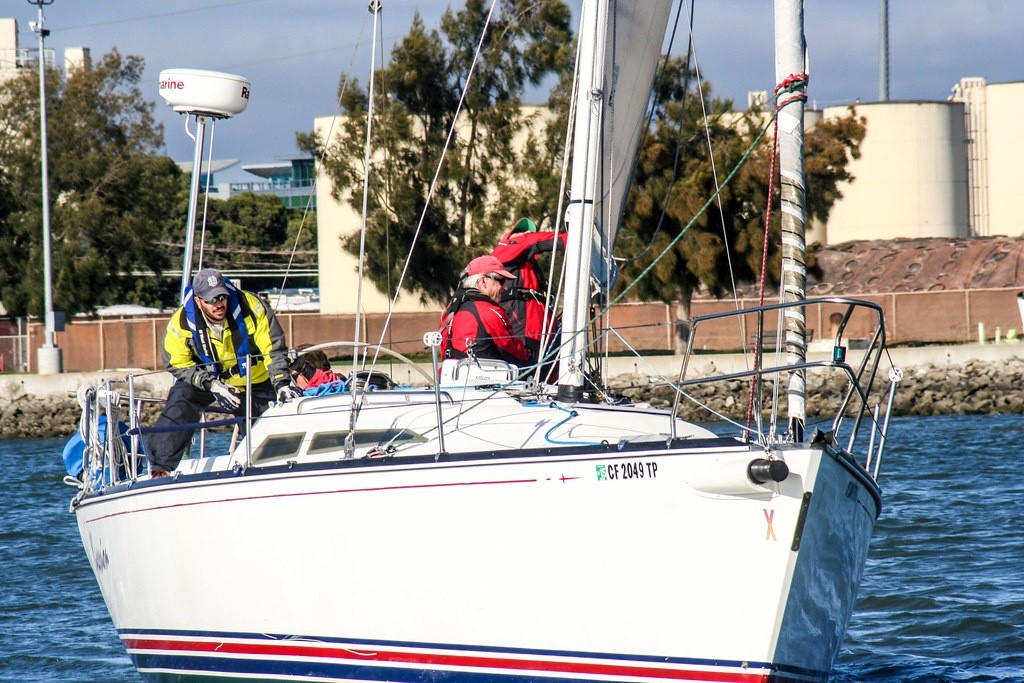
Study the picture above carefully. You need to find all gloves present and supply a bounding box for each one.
[209,378,241,412]
[275,384,301,407]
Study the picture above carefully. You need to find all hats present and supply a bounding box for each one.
[194,267,230,303]
[462,253,517,280]
[500,217,535,241]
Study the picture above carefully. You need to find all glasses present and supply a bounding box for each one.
[485,274,505,285]
[205,294,226,305]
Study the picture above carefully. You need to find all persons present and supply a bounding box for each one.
[436,255,557,388]
[489,217,567,388]
[288,344,347,397]
[146,268,304,481]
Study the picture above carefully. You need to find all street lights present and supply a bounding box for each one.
[26,1,66,375]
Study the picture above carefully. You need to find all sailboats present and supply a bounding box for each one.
[65,0,898,683]
